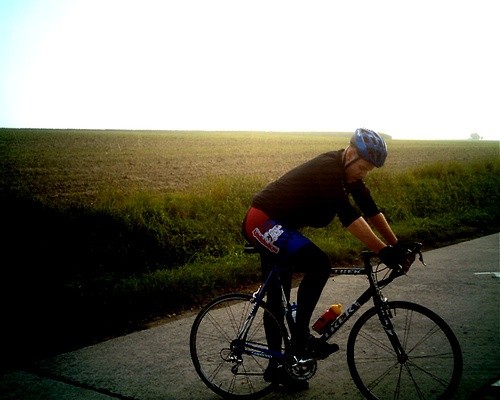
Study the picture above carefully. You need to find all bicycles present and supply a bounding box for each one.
[189,242,464,400]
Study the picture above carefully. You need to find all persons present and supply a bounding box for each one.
[241,127,415,392]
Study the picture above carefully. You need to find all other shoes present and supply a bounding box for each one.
[263,365,310,392]
[287,336,338,358]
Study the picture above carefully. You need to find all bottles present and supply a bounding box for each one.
[312,303,342,335]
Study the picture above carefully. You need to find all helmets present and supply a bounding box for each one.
[349,128,388,168]
